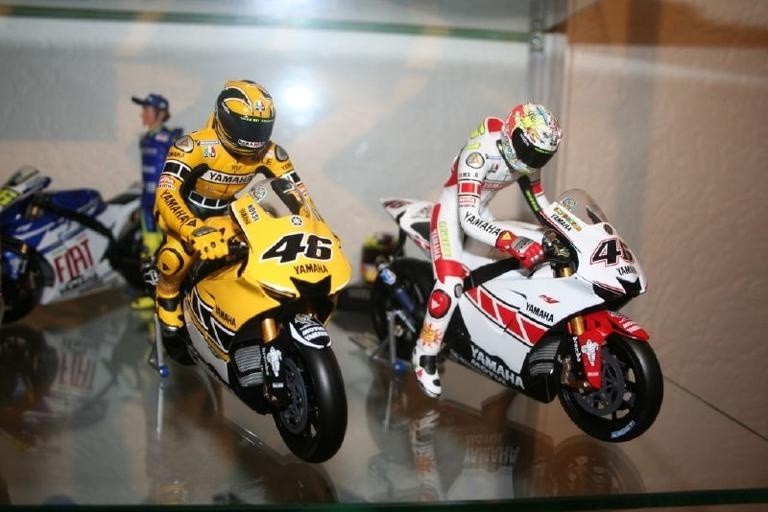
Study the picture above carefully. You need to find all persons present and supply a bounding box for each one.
[152,79,311,338]
[408,410,444,503]
[411,102,563,398]
[130,94,184,310]
